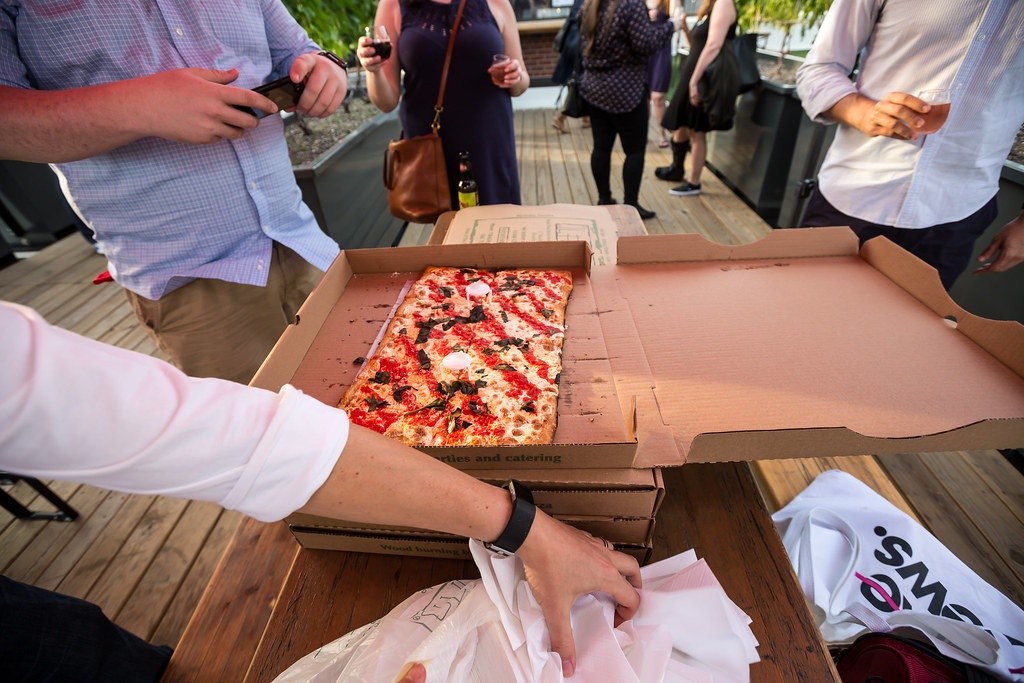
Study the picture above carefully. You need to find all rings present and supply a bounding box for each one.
[600,536,608,547]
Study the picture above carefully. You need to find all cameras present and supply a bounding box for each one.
[232,75,304,119]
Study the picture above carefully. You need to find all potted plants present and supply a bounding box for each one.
[706,0,833,229]
[278,0,406,250]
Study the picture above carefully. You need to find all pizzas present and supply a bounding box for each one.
[342,264,573,446]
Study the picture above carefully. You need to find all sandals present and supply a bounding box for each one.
[580,120,591,128]
[551,116,571,133]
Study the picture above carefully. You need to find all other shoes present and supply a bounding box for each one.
[624,202,656,219]
[597,198,616,205]
[658,140,669,148]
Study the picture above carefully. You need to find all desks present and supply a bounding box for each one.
[240,207,846,683]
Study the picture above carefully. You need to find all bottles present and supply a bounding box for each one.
[457,150,480,211]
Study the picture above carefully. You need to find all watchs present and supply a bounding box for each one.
[318,50,348,71]
[484,479,535,558]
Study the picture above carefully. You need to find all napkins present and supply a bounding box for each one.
[468,536,761,683]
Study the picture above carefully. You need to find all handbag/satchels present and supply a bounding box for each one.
[269,577,499,683]
[561,80,593,118]
[698,33,762,94]
[382,133,452,224]
[552,17,572,53]
[769,469,1024,683]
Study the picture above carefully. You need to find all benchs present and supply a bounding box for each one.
[753,454,921,525]
[158,517,299,683]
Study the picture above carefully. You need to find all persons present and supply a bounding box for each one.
[553,0,739,220]
[357,0,530,226]
[795,0,1024,294]
[0,0,346,384]
[0,301,642,683]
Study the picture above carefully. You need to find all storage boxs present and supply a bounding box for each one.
[246,226,1024,566]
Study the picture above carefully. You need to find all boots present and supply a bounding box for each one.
[655,137,691,182]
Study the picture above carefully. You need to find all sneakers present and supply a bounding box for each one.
[668,182,701,195]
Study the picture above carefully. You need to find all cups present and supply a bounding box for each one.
[491,54,510,88]
[909,89,951,134]
[365,25,392,60]
[673,5,685,20]
